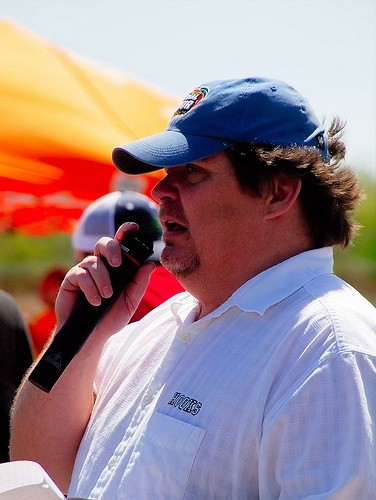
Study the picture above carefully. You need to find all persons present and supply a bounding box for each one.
[0,288,30,462]
[7,78,376,500]
[27,191,185,358]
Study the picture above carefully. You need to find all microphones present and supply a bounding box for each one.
[30,229,154,392]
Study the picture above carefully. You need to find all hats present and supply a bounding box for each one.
[112,76,331,175]
[71,188,169,262]
